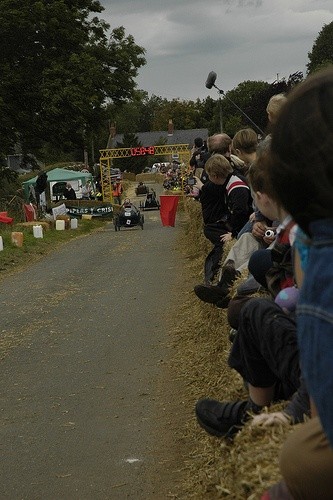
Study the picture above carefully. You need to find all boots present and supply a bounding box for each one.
[194,268,235,309]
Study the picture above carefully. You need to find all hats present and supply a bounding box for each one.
[116,177,121,181]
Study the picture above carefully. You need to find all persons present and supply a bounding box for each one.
[119,205,136,216]
[155,66,333,500]
[22,199,38,222]
[113,178,123,205]
[147,195,154,201]
[138,183,143,187]
[64,183,76,200]
[78,167,107,201]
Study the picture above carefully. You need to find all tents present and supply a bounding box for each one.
[22,168,92,209]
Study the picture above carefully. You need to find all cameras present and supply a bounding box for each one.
[264,226,277,237]
[187,178,196,185]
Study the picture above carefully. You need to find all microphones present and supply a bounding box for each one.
[206,71,217,89]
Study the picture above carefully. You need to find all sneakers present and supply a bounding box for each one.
[195,397,247,442]
